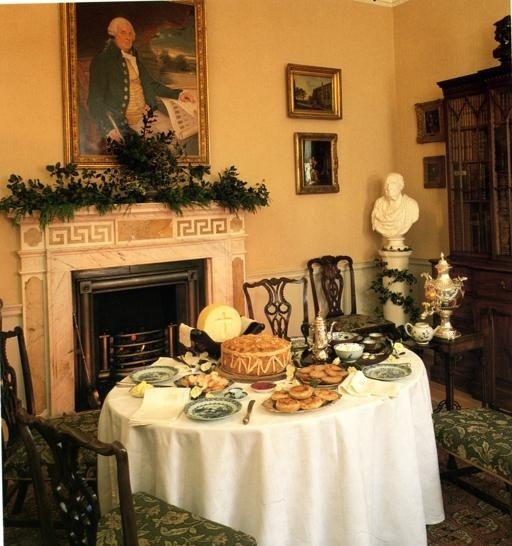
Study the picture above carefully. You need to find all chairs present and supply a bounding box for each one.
[0,326,102,515]
[432,307,512,515]
[308,255,394,334]
[17,407,257,546]
[243,277,308,337]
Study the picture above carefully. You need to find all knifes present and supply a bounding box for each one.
[243,400,256,425]
[115,381,174,388]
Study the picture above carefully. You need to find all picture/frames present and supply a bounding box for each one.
[59,0,209,169]
[287,63,342,119]
[294,132,339,195]
[415,98,446,144]
[423,155,446,188]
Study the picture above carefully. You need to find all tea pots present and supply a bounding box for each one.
[306,311,337,362]
[404,322,441,345]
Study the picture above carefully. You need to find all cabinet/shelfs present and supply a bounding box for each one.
[429,65,512,414]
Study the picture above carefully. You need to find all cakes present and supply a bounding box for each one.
[221,334,293,376]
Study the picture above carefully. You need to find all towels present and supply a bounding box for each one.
[338,372,400,399]
[127,387,190,428]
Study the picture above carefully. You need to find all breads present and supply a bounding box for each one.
[271,385,338,411]
[298,364,347,384]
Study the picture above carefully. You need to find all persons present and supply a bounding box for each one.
[86,16,194,144]
[371,173,420,238]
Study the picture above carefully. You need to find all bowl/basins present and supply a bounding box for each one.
[362,340,376,349]
[228,387,243,399]
[331,332,359,342]
[333,342,365,364]
[369,332,383,341]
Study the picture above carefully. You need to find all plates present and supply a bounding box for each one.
[363,363,412,382]
[224,391,248,402]
[129,365,179,384]
[250,383,277,392]
[261,391,341,414]
[184,397,242,421]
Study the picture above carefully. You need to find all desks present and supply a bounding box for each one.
[414,326,486,412]
